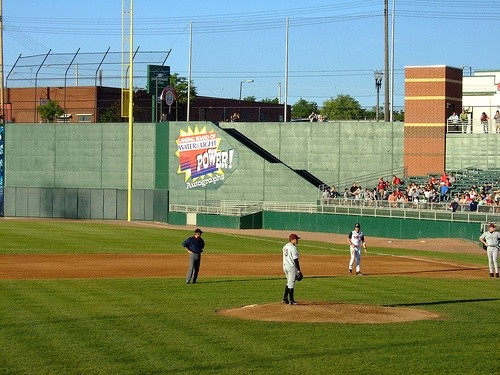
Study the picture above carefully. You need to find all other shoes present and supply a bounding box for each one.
[282,300,289,304]
[348,270,352,274]
[289,301,299,305]
[490,273,494,278]
[496,273,499,278]
[355,272,362,275]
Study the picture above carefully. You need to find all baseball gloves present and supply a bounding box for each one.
[296,273,303,281]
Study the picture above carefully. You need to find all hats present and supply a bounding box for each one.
[355,223,360,228]
[441,182,445,184]
[195,229,203,233]
[289,234,300,239]
[490,223,495,228]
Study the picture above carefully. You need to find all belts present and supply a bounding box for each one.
[488,245,495,247]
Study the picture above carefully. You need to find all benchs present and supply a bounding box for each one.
[368,167,500,210]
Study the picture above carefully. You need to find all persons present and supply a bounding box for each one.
[181,229,205,284]
[308,111,324,122]
[479,223,500,278]
[450,182,500,213]
[460,110,468,133]
[226,112,240,122]
[449,111,459,133]
[282,234,303,305]
[494,111,500,133]
[405,172,456,209]
[322,175,405,208]
[159,112,167,122]
[347,223,367,275]
[481,112,489,133]
